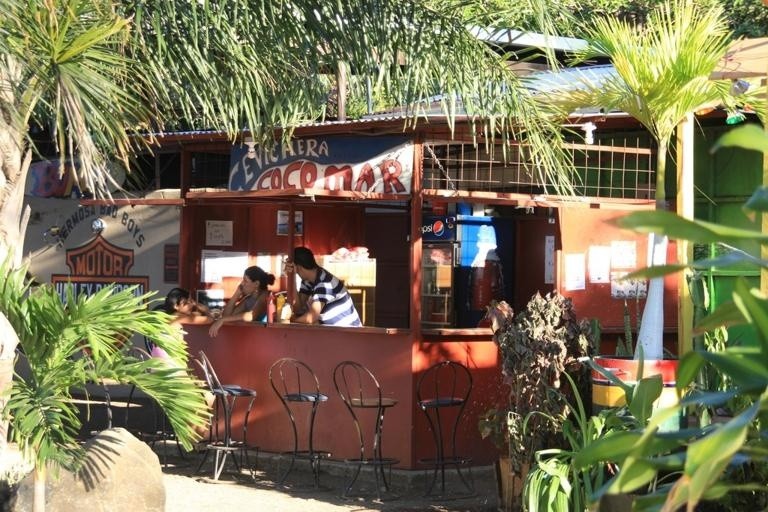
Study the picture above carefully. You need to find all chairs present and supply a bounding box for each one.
[268,354,333,493]
[334,356,402,498]
[416,359,475,501]
[84,339,257,485]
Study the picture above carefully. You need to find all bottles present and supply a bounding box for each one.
[266,290,291,325]
[467,223,506,330]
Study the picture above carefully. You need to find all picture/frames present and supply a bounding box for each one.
[276,209,304,237]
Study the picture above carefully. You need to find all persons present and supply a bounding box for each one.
[150,288,215,437]
[284,246,363,327]
[478,299,514,334]
[208,266,275,338]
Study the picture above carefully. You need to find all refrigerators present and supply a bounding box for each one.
[421,216,512,331]
